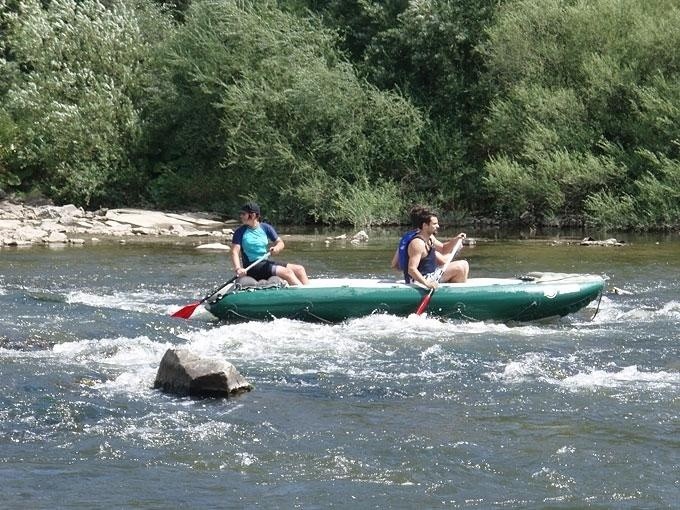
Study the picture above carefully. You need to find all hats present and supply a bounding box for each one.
[238,202,260,214]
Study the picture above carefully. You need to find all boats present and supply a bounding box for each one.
[203,271,611,326]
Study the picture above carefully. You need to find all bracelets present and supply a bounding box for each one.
[235,267,240,272]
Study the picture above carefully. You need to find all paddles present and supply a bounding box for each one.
[416,239,462,315]
[171,251,273,319]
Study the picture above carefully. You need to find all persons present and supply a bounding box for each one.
[391,205,469,283]
[230,203,310,287]
[405,213,465,291]
